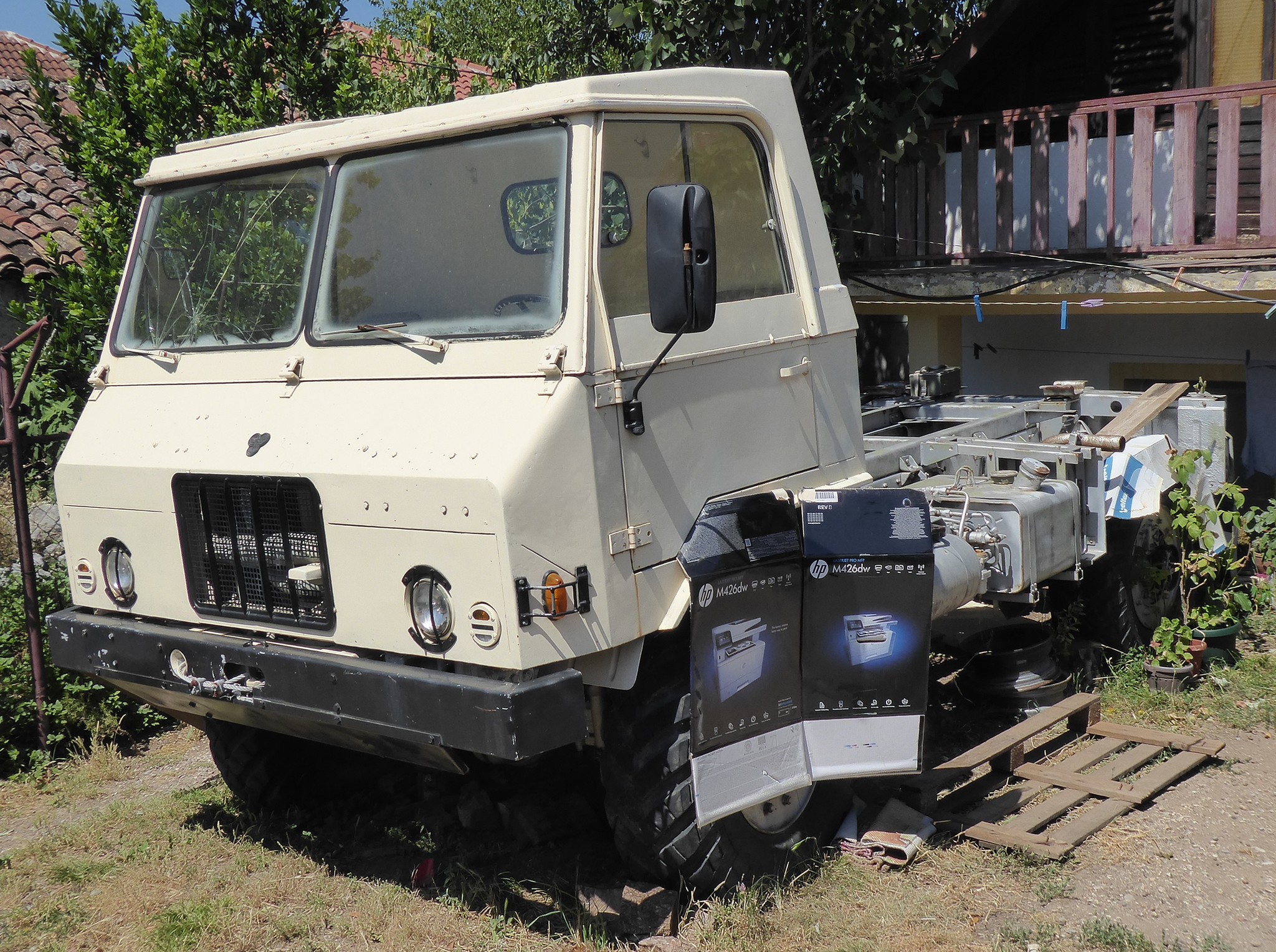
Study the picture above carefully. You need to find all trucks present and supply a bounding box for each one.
[39,64,1219,907]
